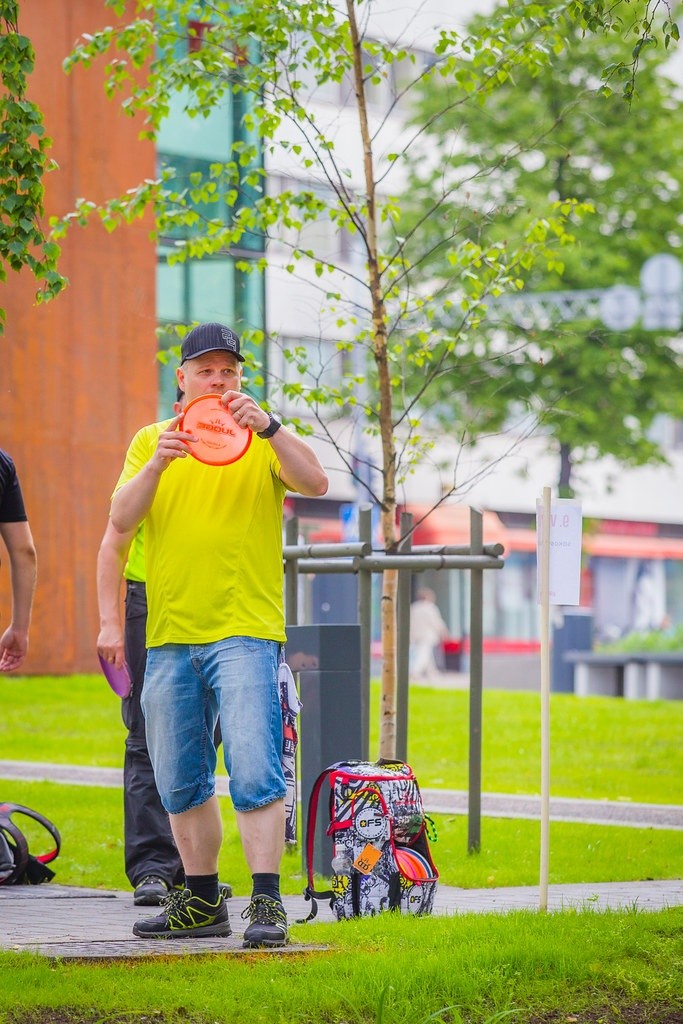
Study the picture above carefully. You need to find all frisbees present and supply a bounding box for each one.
[395,846,433,879]
[179,393,253,467]
[97,652,134,700]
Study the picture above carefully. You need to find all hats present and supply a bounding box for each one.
[180,322,246,366]
[176,384,184,402]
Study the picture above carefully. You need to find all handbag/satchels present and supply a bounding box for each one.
[275,645,304,844]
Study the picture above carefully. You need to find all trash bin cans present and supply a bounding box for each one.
[551,605,592,694]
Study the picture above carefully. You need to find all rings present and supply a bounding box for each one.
[236,410,243,418]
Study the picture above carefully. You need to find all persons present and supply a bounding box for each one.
[409,587,450,680]
[109,322,328,945]
[0,454,37,671]
[94,384,232,905]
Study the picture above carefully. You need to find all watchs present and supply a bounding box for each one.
[257,411,281,439]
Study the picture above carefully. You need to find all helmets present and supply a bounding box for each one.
[0,801,59,885]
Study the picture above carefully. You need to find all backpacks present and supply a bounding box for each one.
[295,757,439,922]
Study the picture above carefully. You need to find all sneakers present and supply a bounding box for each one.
[132,889,233,938]
[173,868,233,899]
[133,876,170,905]
[240,894,289,947]
